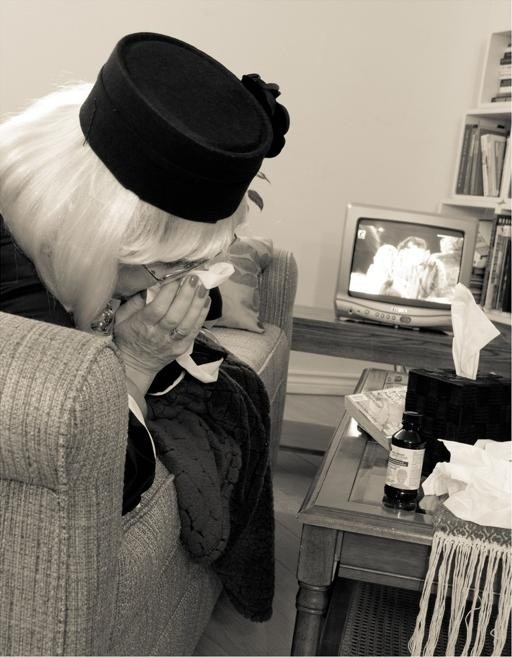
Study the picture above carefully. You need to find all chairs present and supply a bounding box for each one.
[0,224,297,656]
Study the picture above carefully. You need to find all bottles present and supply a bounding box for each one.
[384,409,424,516]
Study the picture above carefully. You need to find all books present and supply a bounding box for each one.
[457,41,512,321]
[342,384,409,450]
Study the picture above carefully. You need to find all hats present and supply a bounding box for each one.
[78,33,289,223]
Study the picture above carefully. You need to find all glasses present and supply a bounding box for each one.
[142,231,238,289]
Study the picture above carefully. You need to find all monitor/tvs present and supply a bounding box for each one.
[334,203,477,330]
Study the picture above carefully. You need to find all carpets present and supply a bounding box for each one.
[289,575,511,656]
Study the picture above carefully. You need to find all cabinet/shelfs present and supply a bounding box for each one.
[439,20,511,321]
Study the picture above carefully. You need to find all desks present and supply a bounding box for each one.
[286,362,510,652]
[281,300,511,377]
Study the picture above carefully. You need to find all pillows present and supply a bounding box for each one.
[201,233,272,329]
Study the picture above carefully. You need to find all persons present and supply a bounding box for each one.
[391,236,443,299]
[0,26,287,514]
[414,236,463,300]
[347,221,397,296]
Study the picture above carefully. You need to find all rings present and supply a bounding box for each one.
[170,327,189,343]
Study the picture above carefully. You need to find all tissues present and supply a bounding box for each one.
[403,281,512,481]
